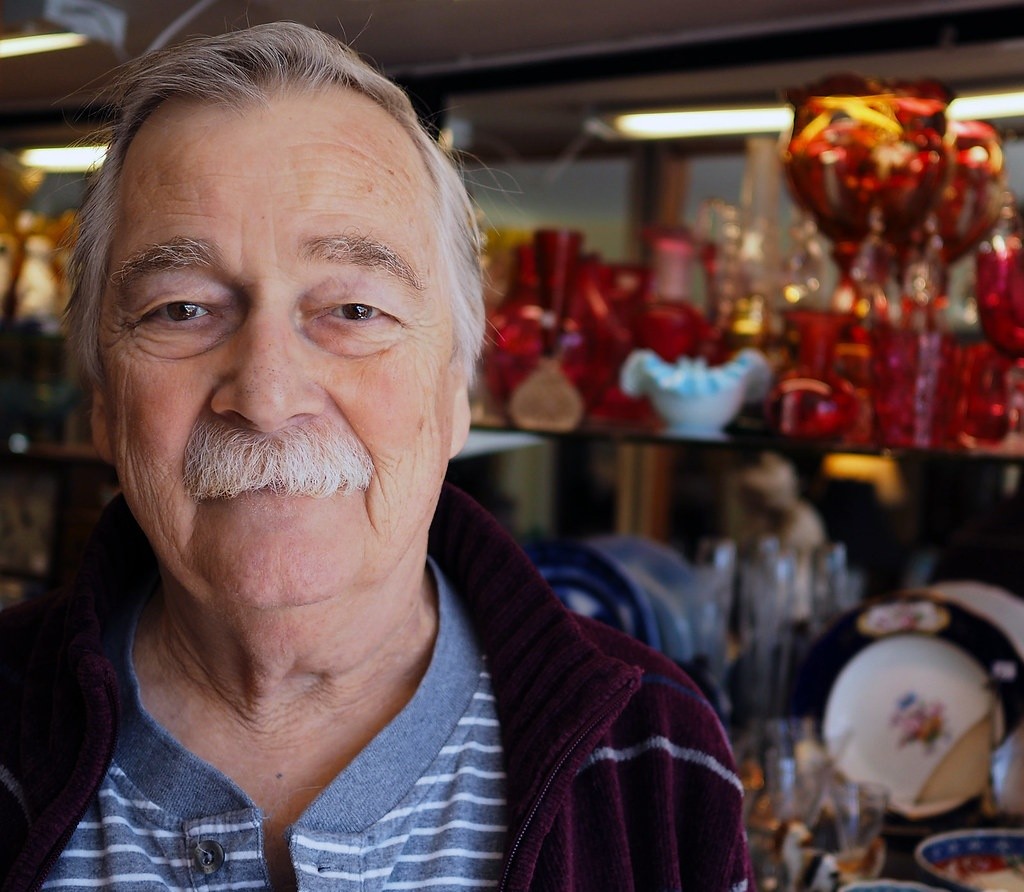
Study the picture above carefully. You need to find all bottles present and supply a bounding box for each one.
[510,358,582,432]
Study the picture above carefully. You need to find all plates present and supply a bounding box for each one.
[837,877,947,892]
[785,592,1023,856]
[913,827,1024,892]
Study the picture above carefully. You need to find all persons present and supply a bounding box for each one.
[0,22,754,892]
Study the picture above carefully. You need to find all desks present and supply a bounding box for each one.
[448,432,552,536]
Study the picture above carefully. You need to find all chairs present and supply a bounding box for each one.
[543,537,726,705]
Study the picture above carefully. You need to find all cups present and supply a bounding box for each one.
[685,539,885,892]
